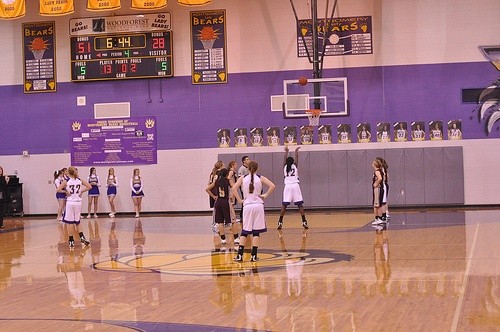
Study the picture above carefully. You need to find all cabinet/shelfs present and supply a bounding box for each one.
[3,174,25,218]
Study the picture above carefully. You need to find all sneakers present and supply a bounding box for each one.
[81,237,90,246]
[277,222,282,229]
[250,255,259,261]
[69,241,74,247]
[302,221,309,229]
[233,255,243,261]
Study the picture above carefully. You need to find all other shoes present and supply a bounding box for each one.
[372,215,390,225]
[135,214,139,218]
[80,216,84,219]
[94,214,98,218]
[87,215,91,219]
[108,212,116,218]
[236,219,240,223]
[220,240,226,245]
[212,224,218,228]
[234,239,240,245]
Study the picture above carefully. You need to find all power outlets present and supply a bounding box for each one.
[48,180,52,184]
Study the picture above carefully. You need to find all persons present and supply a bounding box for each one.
[208,161,240,227]
[268,130,279,143]
[106,168,119,218]
[238,156,251,222]
[0,166,9,228]
[129,169,145,217]
[54,168,84,220]
[277,146,309,229]
[300,128,312,142]
[205,168,240,245]
[339,122,461,140]
[86,167,101,218]
[319,127,331,140]
[285,129,297,142]
[250,130,263,144]
[235,129,247,144]
[372,157,391,225]
[218,131,230,144]
[57,167,92,247]
[231,161,275,261]
